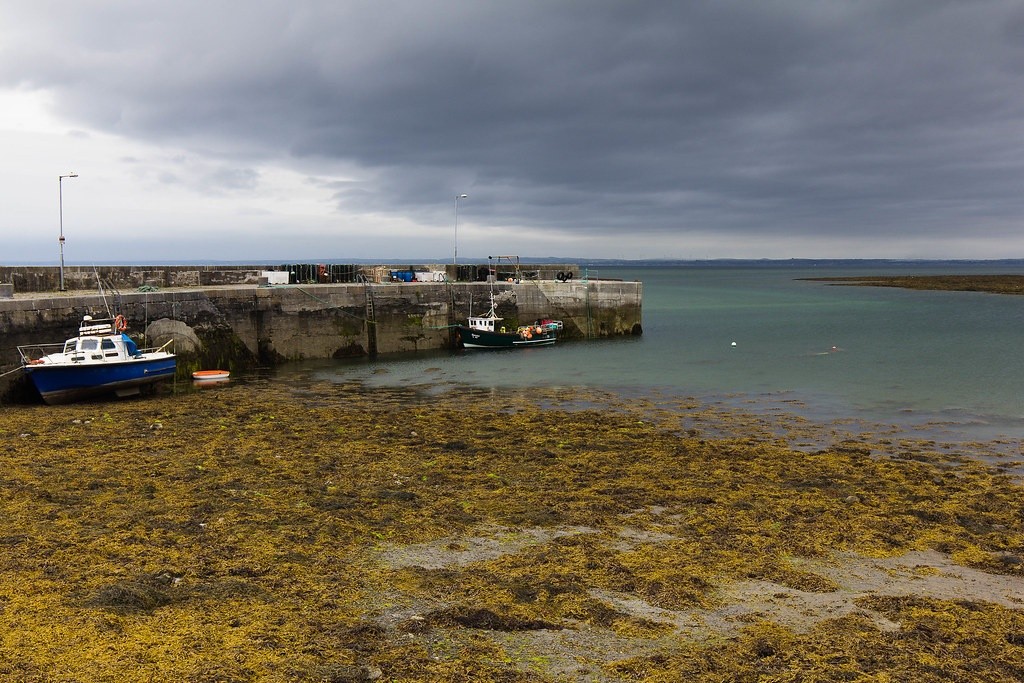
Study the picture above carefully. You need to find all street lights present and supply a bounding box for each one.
[59,174,79,291]
[454,193,468,265]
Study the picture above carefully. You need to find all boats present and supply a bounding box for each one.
[192,369,230,380]
[14,265,178,405]
[457,291,563,352]
[193,377,232,388]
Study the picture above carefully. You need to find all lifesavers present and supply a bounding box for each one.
[557,272,564,280]
[562,275,567,281]
[566,271,573,279]
[115,315,127,331]
[31,360,45,365]
